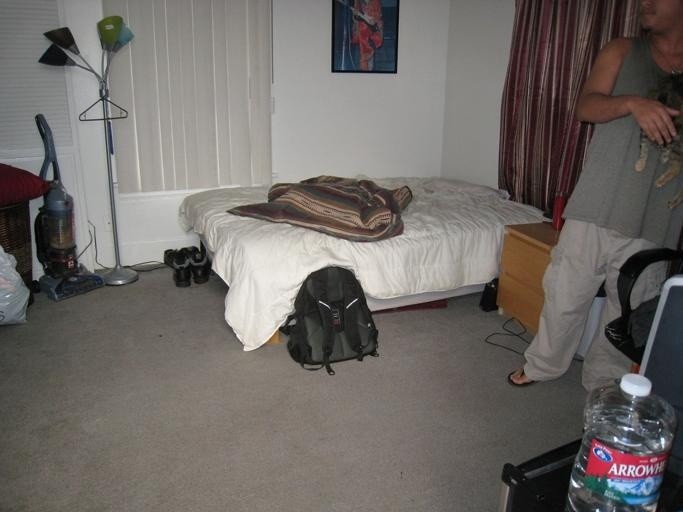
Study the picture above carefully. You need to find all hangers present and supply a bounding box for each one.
[78,81,129,122]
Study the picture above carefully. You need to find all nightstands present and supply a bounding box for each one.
[496,222,560,336]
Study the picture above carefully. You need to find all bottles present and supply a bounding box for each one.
[568,372,677,512]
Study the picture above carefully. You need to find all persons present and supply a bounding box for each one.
[507,1,683,395]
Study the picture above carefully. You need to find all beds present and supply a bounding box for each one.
[184,177,552,331]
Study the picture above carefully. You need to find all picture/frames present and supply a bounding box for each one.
[332,0,400,74]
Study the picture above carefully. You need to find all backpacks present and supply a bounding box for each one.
[279,267,378,375]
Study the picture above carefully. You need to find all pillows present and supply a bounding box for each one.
[421,174,511,203]
[1,161,49,206]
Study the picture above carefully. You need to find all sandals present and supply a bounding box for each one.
[506,365,535,387]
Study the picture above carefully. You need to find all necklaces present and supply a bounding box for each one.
[649,34,682,75]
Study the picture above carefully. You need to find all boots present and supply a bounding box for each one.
[172,247,190,287]
[189,240,208,284]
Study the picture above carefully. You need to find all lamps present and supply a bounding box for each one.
[38,16,138,288]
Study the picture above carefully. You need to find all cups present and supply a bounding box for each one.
[553,190,565,230]
